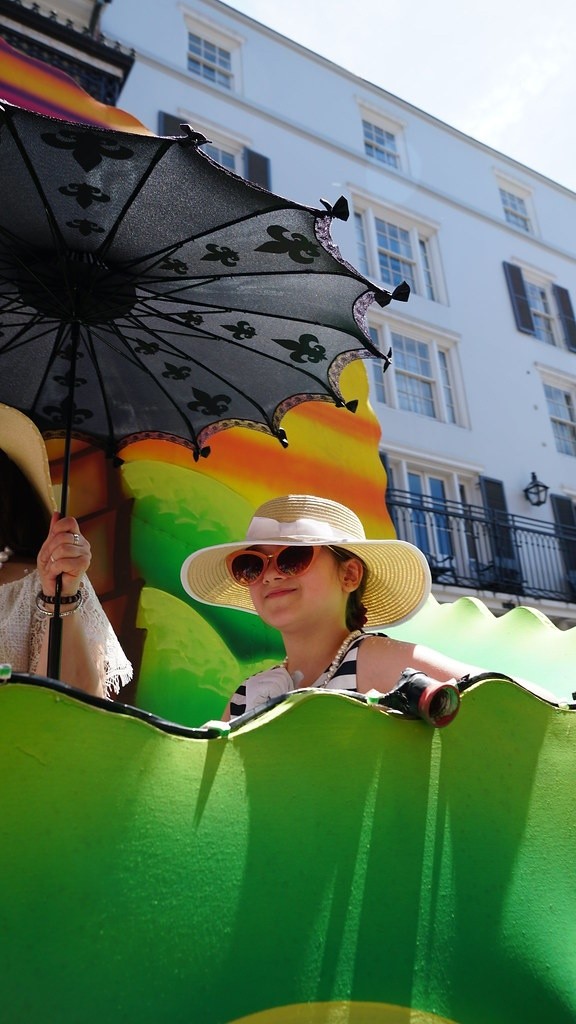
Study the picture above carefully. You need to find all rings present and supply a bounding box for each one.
[50,555,56,562]
[73,533,79,544]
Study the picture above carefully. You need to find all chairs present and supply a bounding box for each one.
[564,570,576,602]
[470,561,528,595]
[417,550,459,586]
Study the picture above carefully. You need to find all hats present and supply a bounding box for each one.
[0,403,58,517]
[180,494,432,631]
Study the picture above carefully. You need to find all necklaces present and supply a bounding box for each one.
[280,629,362,688]
[0,548,11,567]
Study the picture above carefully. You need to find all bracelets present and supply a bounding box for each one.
[35,590,84,617]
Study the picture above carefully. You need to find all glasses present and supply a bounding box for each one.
[225,543,345,586]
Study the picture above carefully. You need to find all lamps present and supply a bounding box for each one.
[522,471,550,507]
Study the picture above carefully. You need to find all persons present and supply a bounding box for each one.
[180,495,476,721]
[0,403,134,700]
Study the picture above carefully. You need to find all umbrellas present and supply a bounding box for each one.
[0,100,411,684]
